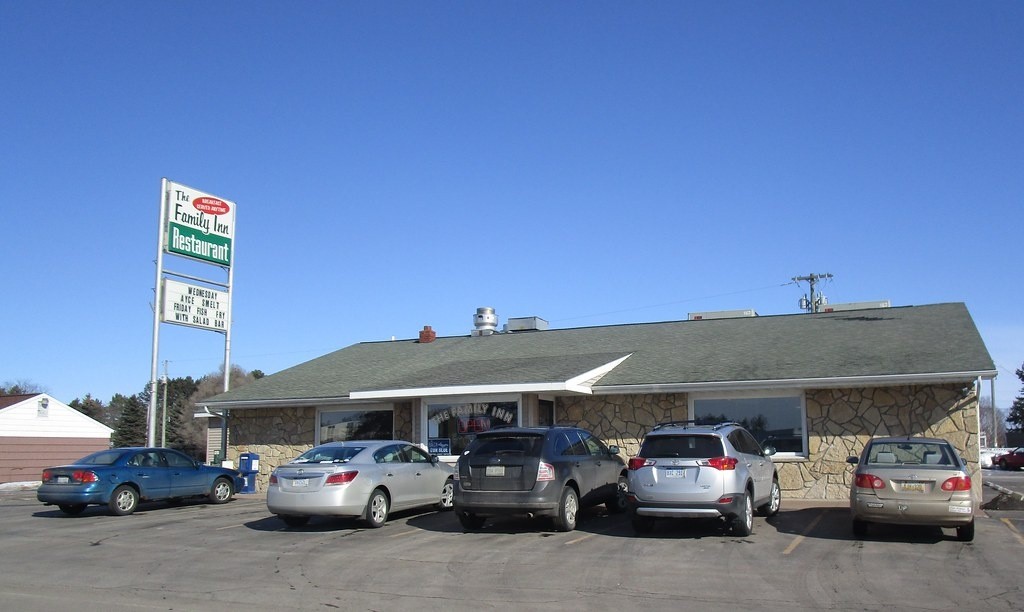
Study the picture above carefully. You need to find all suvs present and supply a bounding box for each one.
[627,420,782,537]
[452,424,629,531]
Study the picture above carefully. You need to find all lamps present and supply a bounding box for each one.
[38,398,49,409]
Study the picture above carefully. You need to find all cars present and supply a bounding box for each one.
[844,433,976,541]
[998,445,1024,470]
[264,439,456,528]
[35,447,247,517]
